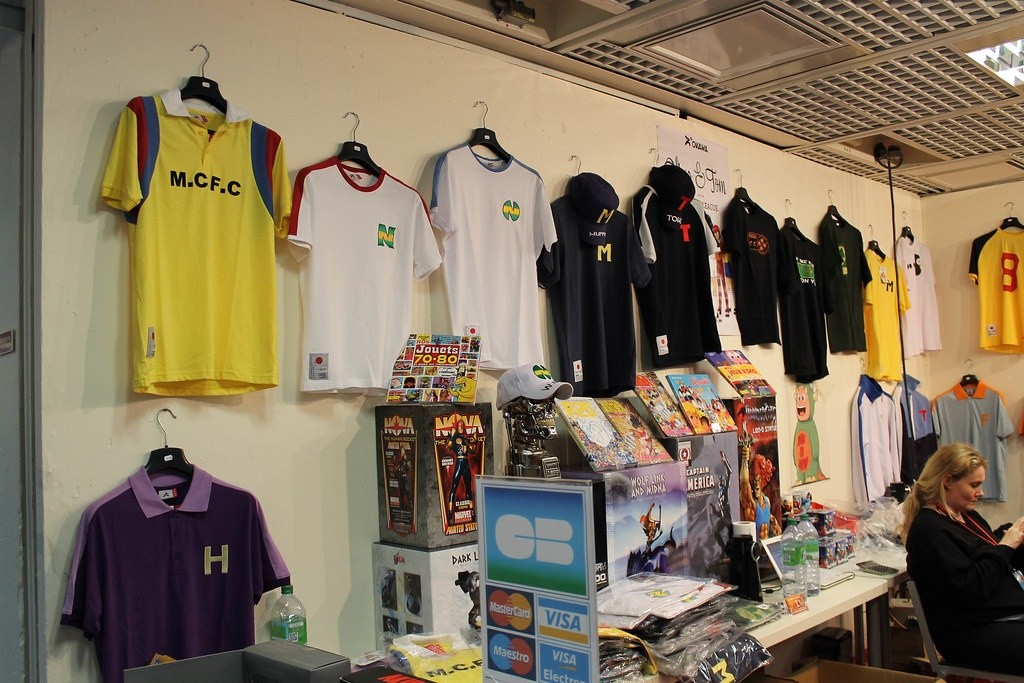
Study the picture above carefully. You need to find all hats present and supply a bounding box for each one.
[651,165,696,231]
[496,363,574,411]
[569,172,620,246]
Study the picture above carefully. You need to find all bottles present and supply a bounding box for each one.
[271,585,307,646]
[796,514,820,598]
[781,518,807,601]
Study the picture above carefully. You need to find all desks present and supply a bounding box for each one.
[641,576,894,683]
[854,547,912,668]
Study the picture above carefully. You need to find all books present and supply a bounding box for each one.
[704,349,778,398]
[554,372,739,473]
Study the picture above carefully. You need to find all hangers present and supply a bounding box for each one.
[548,152,635,216]
[950,356,986,390]
[296,112,429,199]
[780,199,813,235]
[860,225,889,254]
[438,98,547,169]
[636,149,711,199]
[981,202,1024,243]
[897,209,926,244]
[79,406,284,512]
[854,358,896,389]
[819,189,862,225]
[125,43,266,134]
[724,169,772,208]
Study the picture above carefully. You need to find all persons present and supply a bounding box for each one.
[901,443,1024,677]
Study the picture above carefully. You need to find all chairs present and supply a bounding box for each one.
[907,577,1024,683]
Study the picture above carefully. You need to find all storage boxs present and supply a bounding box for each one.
[241,638,351,683]
[372,334,803,648]
[774,652,945,683]
